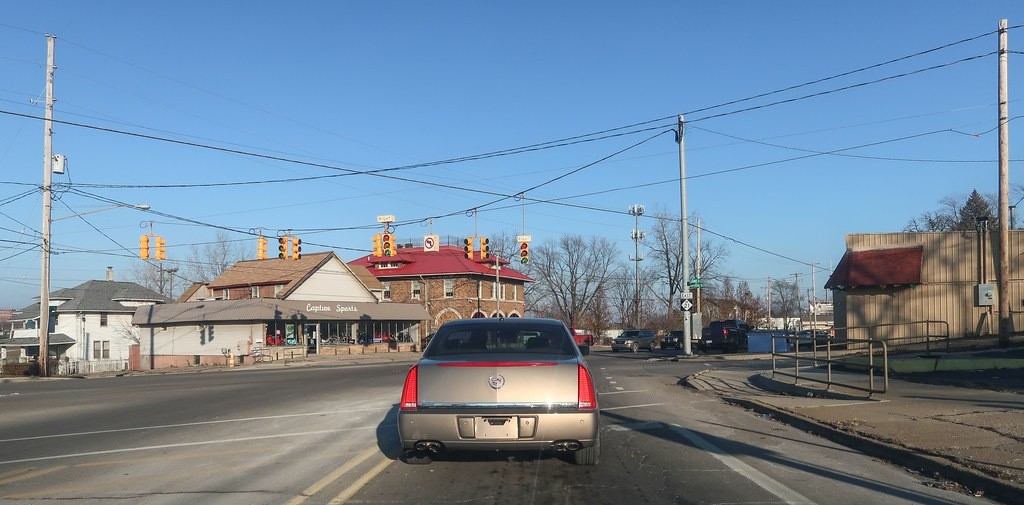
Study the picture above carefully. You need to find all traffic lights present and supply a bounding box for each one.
[155,235,167,260]
[140,236,150,260]
[291,237,302,261]
[278,237,288,260]
[372,234,382,258]
[390,235,397,256]
[520,242,529,265]
[480,237,490,260]
[382,233,393,257]
[258,237,266,262]
[464,237,474,260]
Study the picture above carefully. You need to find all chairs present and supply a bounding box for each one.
[527,337,549,348]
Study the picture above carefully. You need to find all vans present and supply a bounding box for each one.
[610,329,658,353]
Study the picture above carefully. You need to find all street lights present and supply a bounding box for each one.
[627,204,647,331]
[40,204,152,377]
[165,267,179,297]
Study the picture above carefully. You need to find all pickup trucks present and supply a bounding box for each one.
[702,319,755,346]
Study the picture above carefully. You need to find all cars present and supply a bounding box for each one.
[660,330,683,350]
[396,317,601,466]
[792,329,833,349]
